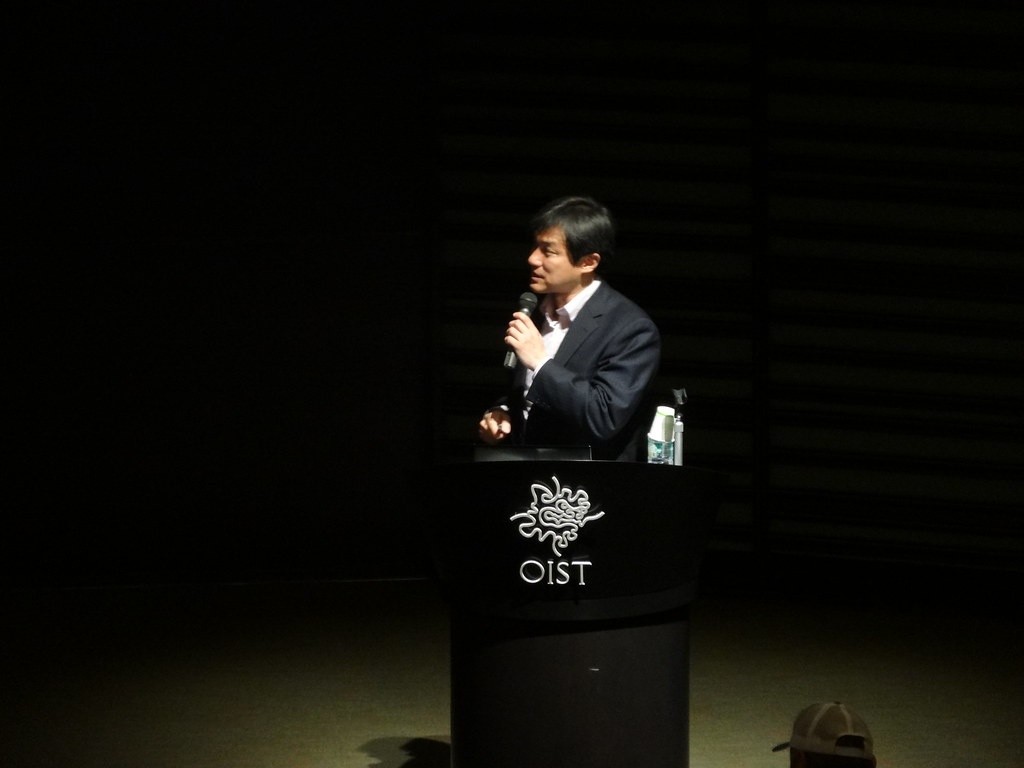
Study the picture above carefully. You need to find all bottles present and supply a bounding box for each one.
[647,436,673,465]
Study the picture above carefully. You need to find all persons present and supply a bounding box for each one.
[772,700,877,768]
[475,193,664,463]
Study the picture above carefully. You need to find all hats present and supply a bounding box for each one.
[790,702,873,759]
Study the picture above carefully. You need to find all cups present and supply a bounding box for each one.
[646,406,675,443]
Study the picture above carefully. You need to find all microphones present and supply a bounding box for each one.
[504,292,537,368]
